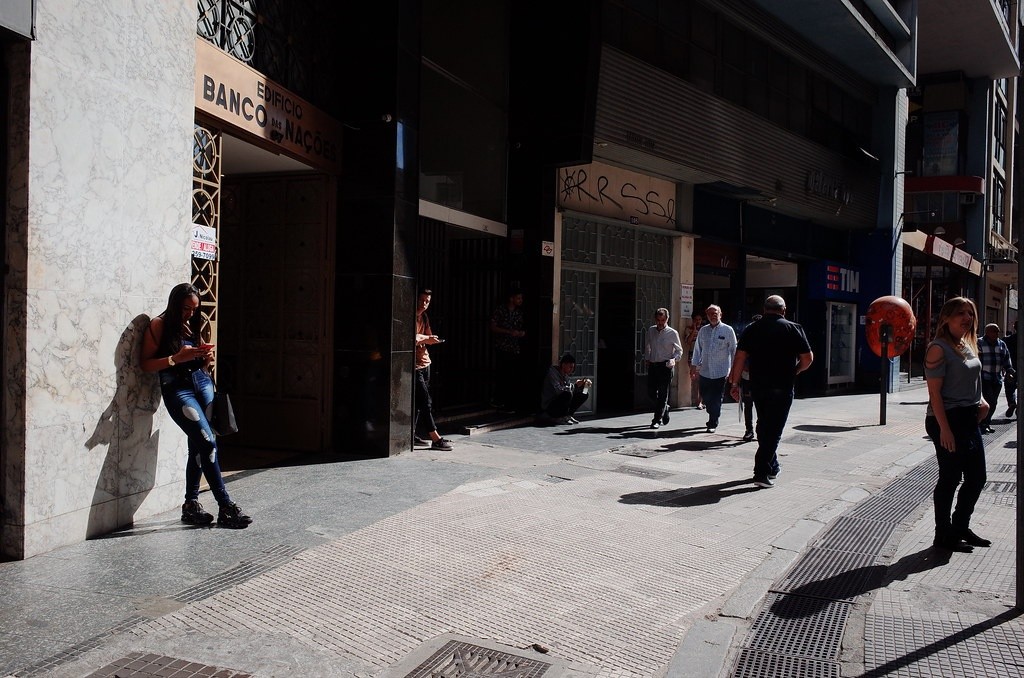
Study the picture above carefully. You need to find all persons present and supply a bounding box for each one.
[686,309,706,410]
[645,308,684,429]
[729,314,762,441]
[140,283,253,523]
[690,304,737,433]
[541,355,593,425]
[924,297,992,550]
[489,287,527,414]
[730,294,814,487]
[976,323,1015,435]
[1001,321,1019,416]
[417,287,452,451]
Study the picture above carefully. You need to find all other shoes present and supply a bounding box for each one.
[980,427,995,433]
[662,405,670,425]
[1006,406,1015,417]
[697,404,703,410]
[707,428,715,432]
[743,431,754,441]
[559,417,579,425]
[702,404,706,408]
[958,529,992,545]
[651,422,659,428]
[933,530,973,551]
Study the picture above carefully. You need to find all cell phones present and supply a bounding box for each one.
[438,340,445,342]
[199,343,215,351]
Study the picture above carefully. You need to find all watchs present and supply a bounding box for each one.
[168,356,175,366]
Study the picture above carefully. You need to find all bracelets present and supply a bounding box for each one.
[732,383,737,385]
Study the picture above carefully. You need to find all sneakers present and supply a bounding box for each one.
[413,436,429,446]
[433,438,454,450]
[182,502,214,523]
[754,477,776,487]
[769,468,781,479]
[217,506,252,524]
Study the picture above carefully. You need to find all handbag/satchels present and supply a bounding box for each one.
[211,393,238,436]
